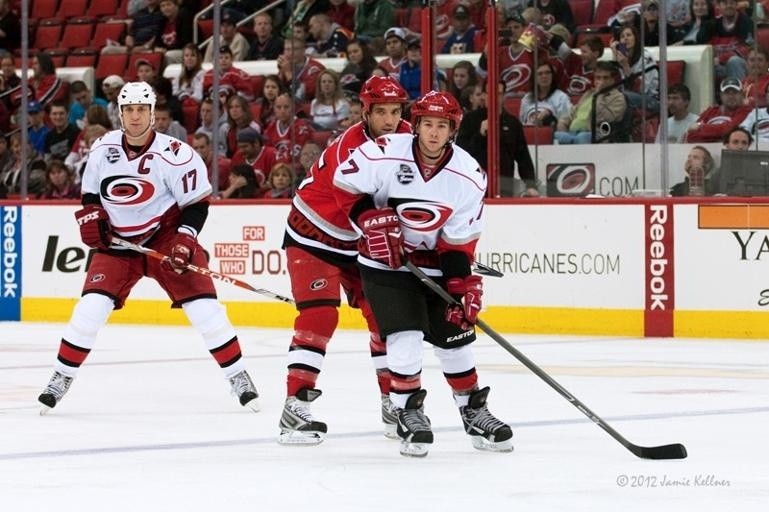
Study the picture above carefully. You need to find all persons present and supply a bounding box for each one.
[1,1,768,202]
[39,80,259,408]
[279,75,431,434]
[332,89,513,444]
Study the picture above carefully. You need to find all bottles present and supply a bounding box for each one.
[689,160,705,196]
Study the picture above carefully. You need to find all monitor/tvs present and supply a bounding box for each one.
[717,148,769,198]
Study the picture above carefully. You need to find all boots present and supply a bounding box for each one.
[388,386,436,444]
[37,368,75,408]
[458,384,516,443]
[380,392,432,427]
[227,368,260,408]
[278,385,329,432]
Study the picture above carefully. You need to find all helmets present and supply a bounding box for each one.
[408,89,466,131]
[357,73,410,114]
[115,80,158,126]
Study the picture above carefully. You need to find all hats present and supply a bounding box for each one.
[407,38,421,50]
[548,23,570,43]
[221,14,234,24]
[26,100,42,114]
[234,126,270,144]
[645,0,660,11]
[382,27,406,43]
[134,58,153,67]
[451,4,472,19]
[504,10,526,25]
[719,76,744,94]
[102,74,125,88]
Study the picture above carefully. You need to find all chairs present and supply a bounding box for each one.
[0,1,769,201]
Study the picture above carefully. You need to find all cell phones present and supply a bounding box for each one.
[617,42,627,55]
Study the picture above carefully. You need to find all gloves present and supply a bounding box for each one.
[159,233,199,272]
[442,273,486,332]
[353,203,409,271]
[73,202,114,253]
